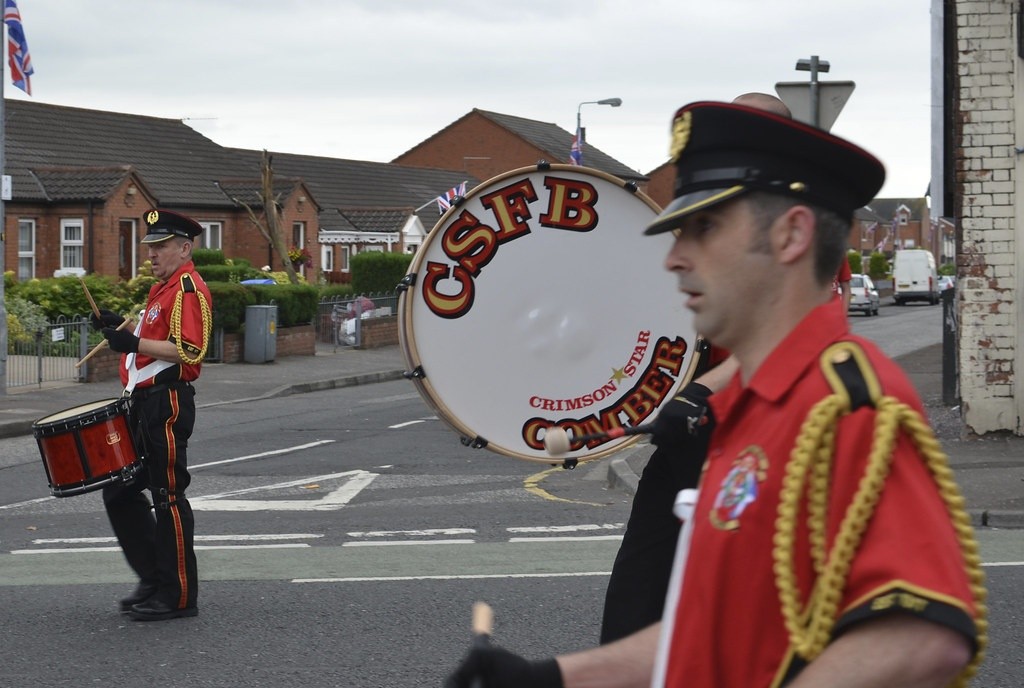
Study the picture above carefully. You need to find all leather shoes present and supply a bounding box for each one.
[119,584,159,610]
[130,598,197,621]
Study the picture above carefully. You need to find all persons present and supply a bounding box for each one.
[444,92,988,688]
[90,209,213,620]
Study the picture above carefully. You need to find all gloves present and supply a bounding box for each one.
[442,642,566,688]
[88,311,126,331]
[646,382,713,466]
[102,328,141,354]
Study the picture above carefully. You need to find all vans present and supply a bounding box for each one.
[892,249,941,305]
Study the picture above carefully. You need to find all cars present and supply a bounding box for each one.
[939,276,953,297]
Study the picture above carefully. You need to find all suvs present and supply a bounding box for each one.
[838,272,880,316]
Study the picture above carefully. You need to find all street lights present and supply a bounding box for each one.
[577,97,622,169]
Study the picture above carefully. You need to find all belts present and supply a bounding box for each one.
[132,381,190,399]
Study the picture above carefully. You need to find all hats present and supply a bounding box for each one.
[140,208,204,244]
[644,102,885,236]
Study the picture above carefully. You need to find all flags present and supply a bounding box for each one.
[568,135,584,165]
[869,225,876,234]
[438,183,466,215]
[3,0,34,95]
[878,239,887,253]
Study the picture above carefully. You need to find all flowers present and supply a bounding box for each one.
[288,245,313,270]
